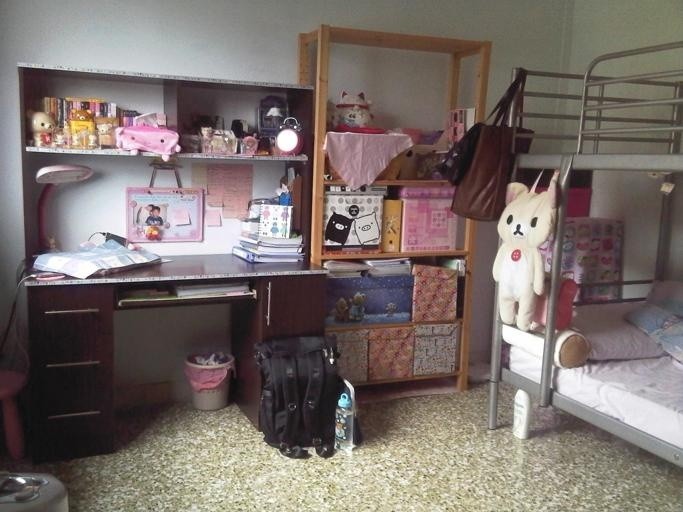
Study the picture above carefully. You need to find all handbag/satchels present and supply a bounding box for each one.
[435,66,524,186]
[449,83,534,223]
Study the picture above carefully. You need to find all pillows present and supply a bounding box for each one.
[533,272,579,333]
[646,281,683,319]
[573,298,667,361]
[540,326,591,371]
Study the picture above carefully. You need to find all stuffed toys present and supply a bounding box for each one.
[492,168,562,333]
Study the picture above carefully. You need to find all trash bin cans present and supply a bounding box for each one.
[185,353,234,412]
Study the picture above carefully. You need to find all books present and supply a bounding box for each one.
[174,280,253,300]
[322,260,371,280]
[364,256,412,278]
[44,94,141,126]
[257,203,293,240]
[231,230,306,265]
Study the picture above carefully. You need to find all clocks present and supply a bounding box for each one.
[274,115,305,157]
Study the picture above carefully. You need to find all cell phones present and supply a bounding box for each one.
[35,272,65,282]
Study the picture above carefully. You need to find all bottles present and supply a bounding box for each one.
[332,392,355,452]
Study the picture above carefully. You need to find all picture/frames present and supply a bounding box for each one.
[258,93,290,139]
[124,185,205,244]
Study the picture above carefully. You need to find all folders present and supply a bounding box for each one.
[232,247,303,263]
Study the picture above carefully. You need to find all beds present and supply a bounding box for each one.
[487,40,683,469]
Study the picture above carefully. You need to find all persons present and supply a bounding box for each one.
[136,204,164,226]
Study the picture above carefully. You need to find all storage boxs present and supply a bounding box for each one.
[369,326,416,381]
[325,273,414,329]
[323,329,368,383]
[412,264,459,324]
[412,323,459,376]
[322,185,388,247]
[399,187,460,254]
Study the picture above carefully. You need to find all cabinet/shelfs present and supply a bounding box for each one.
[15,59,330,465]
[296,30,493,393]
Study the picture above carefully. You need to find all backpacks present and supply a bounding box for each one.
[253,334,363,460]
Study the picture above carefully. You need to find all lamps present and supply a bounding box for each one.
[32,163,95,254]
